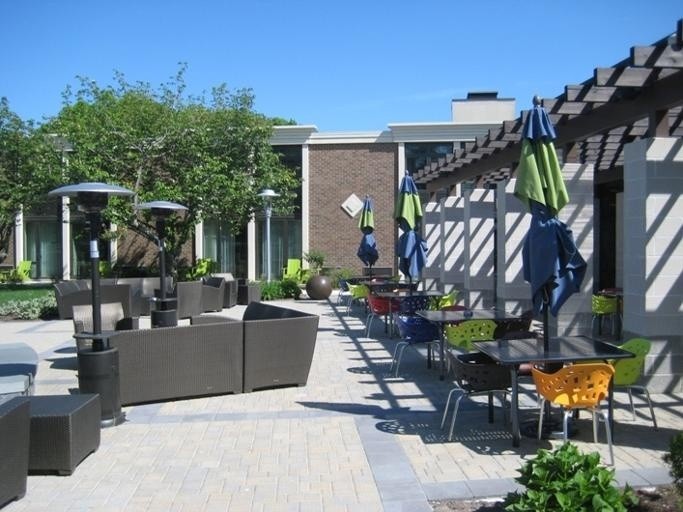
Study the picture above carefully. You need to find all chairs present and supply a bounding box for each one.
[528,362,618,471]
[591,283,623,338]
[336,276,540,382]
[440,349,523,444]
[575,338,658,428]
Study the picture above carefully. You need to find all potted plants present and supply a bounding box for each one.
[304,250,333,299]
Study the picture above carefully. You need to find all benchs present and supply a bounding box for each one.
[52,273,319,403]
[0,396,32,508]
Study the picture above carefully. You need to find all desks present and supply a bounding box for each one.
[471,333,637,448]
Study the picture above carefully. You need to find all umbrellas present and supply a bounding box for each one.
[513,95,588,421]
[357,194,379,283]
[395,169,428,294]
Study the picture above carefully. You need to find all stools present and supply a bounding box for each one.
[27,392,101,475]
